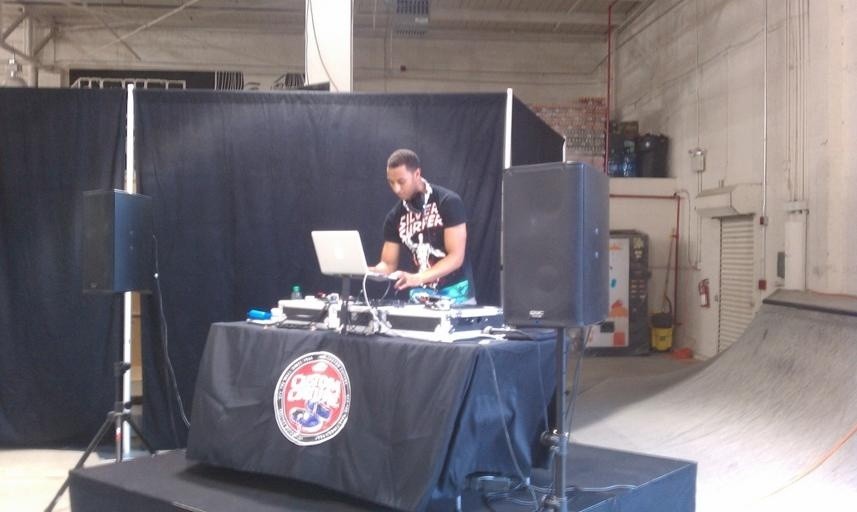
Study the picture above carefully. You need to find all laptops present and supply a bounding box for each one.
[310,230,396,282]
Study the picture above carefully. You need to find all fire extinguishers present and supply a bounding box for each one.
[699,278,709,307]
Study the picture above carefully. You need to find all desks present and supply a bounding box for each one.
[186,316,575,512]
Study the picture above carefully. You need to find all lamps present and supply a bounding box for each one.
[687,147,704,158]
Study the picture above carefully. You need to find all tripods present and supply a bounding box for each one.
[43,293,155,511]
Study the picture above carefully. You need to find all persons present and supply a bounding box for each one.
[366,149,476,307]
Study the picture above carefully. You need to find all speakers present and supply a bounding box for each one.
[81,189,157,295]
[499,162,609,329]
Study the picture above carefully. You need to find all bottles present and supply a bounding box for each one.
[290,286,302,299]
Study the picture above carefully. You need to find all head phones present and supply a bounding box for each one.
[401,177,432,212]
